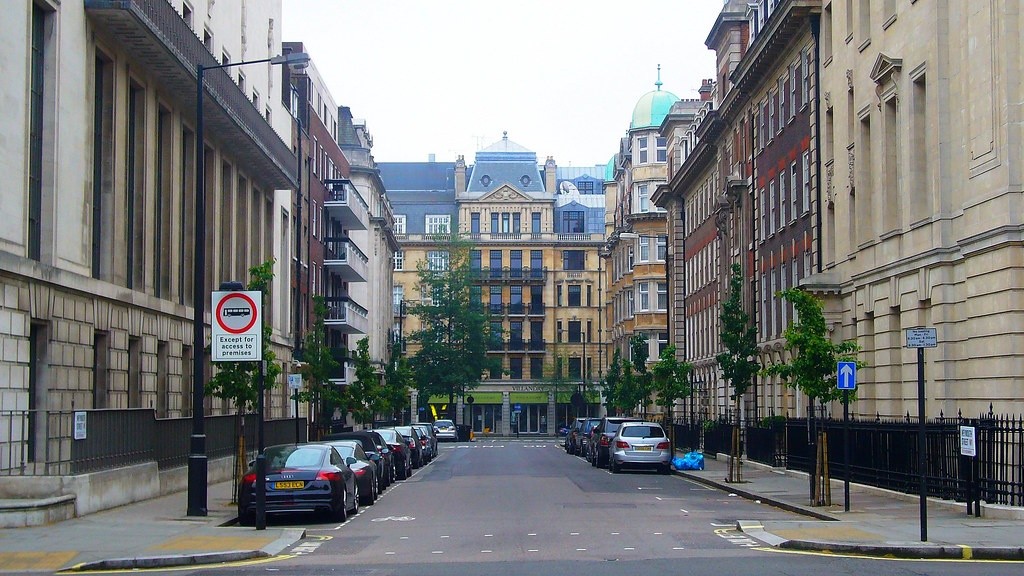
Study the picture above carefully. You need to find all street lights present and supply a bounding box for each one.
[396,298,433,361]
[558,328,587,396]
[185,51,316,525]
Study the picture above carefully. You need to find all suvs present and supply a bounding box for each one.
[590,417,647,468]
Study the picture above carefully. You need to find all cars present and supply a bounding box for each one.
[237,443,360,523]
[565,417,602,457]
[433,419,459,442]
[609,422,672,474]
[408,423,439,465]
[364,429,412,479]
[302,439,378,507]
[384,425,423,470]
[318,431,395,494]
